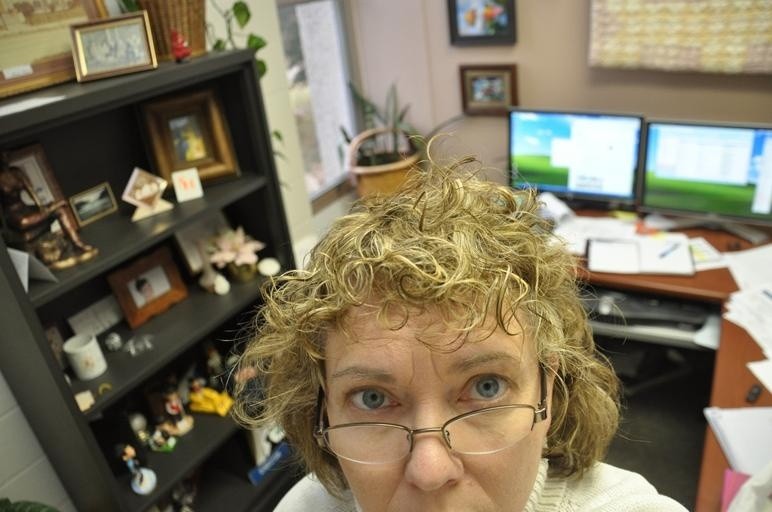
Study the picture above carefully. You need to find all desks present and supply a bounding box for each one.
[572,209,772,512]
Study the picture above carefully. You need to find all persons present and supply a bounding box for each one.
[0,151,92,253]
[213,132,689,512]
[135,278,155,303]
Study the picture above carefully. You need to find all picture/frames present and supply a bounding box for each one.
[68,182,118,227]
[8,143,77,237]
[460,65,516,115]
[134,84,242,197]
[0,0,109,98]
[447,0,516,45]
[107,247,188,329]
[68,9,158,83]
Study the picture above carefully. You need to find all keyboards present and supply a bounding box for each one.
[579,284,708,331]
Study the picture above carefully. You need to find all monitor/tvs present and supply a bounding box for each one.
[639,118,771,245]
[504,106,644,210]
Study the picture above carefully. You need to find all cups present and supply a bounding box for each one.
[62,333,108,381]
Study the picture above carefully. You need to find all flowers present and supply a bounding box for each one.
[210,227,263,269]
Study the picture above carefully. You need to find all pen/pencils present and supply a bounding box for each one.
[661,243,678,257]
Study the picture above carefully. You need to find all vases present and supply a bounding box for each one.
[229,263,256,281]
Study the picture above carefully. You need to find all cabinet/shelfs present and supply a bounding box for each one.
[0,48,307,512]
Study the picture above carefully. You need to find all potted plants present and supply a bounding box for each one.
[338,80,463,197]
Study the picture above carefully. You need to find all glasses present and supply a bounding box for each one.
[314,364,549,468]
